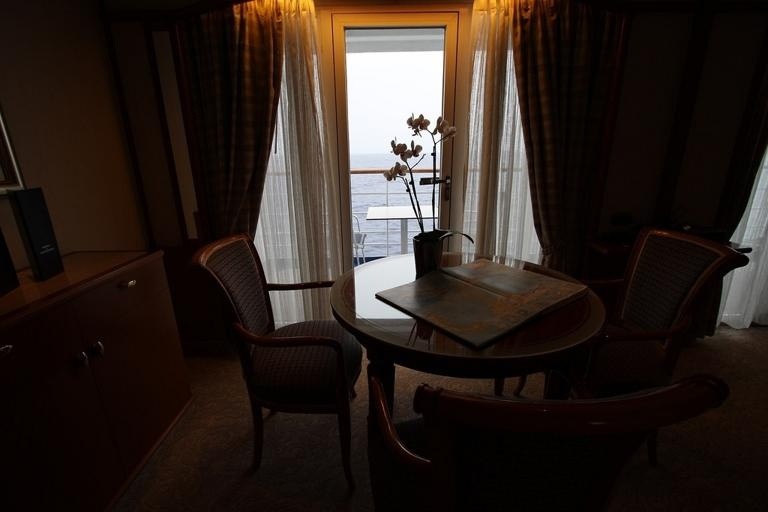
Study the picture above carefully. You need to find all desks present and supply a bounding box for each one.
[366,205,438,255]
[329,254,607,468]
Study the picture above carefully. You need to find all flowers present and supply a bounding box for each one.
[384,115,456,236]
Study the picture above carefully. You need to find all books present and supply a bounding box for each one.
[373,256,589,349]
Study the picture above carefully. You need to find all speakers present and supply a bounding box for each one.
[8,185,64,282]
[0,230,21,297]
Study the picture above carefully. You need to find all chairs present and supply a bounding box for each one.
[352,214,367,266]
[191,233,363,494]
[368,369,731,511]
[515,226,750,397]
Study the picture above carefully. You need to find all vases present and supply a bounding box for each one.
[410,230,476,280]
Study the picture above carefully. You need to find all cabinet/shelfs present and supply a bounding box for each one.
[0,248,199,512]
